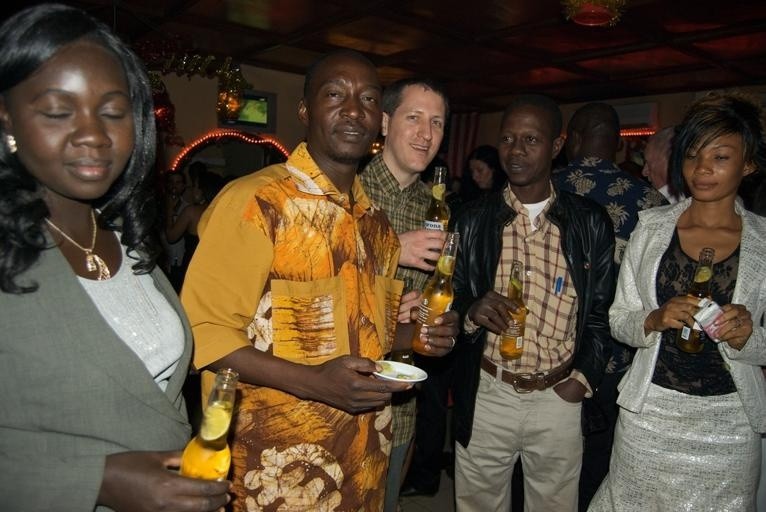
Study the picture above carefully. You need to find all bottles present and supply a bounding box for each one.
[180,369,239,480]
[411,232,461,357]
[499,260,525,359]
[424,166,450,235]
[676,248,715,354]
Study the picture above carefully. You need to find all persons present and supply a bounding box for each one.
[550,103,672,512]
[180,161,207,210]
[643,128,691,205]
[0,5,233,511]
[165,168,226,275]
[159,171,186,269]
[462,144,506,205]
[357,80,459,511]
[443,96,616,512]
[420,143,483,210]
[176,52,402,511]
[588,98,766,512]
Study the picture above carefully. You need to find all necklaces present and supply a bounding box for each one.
[45,207,111,282]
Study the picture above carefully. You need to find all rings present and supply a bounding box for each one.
[450,336,456,347]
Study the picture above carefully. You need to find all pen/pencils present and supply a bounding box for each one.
[554,277,562,297]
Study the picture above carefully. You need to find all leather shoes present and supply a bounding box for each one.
[399,478,440,499]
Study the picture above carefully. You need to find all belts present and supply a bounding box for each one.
[482,361,575,394]
